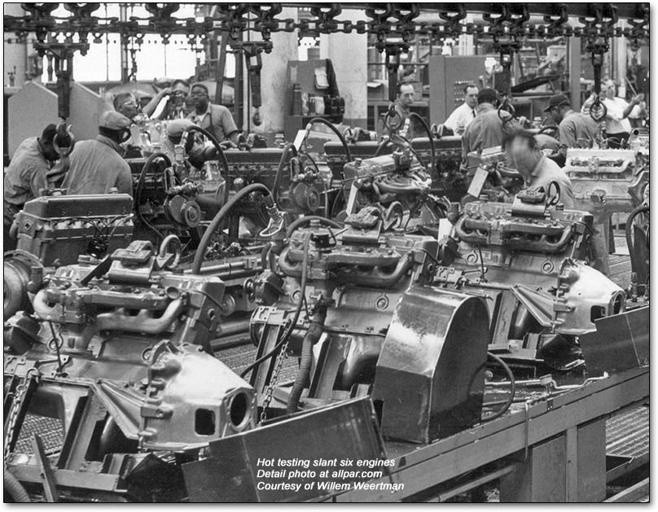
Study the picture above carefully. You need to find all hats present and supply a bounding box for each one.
[477,88,501,106]
[543,94,571,111]
[167,119,200,137]
[99,111,133,131]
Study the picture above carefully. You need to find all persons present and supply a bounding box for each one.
[597,76,645,150]
[501,132,576,210]
[441,84,480,136]
[58,109,133,195]
[383,81,416,138]
[113,78,246,217]
[543,92,603,149]
[4,121,78,229]
[462,87,526,188]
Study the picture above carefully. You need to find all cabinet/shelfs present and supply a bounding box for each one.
[429,55,495,127]
